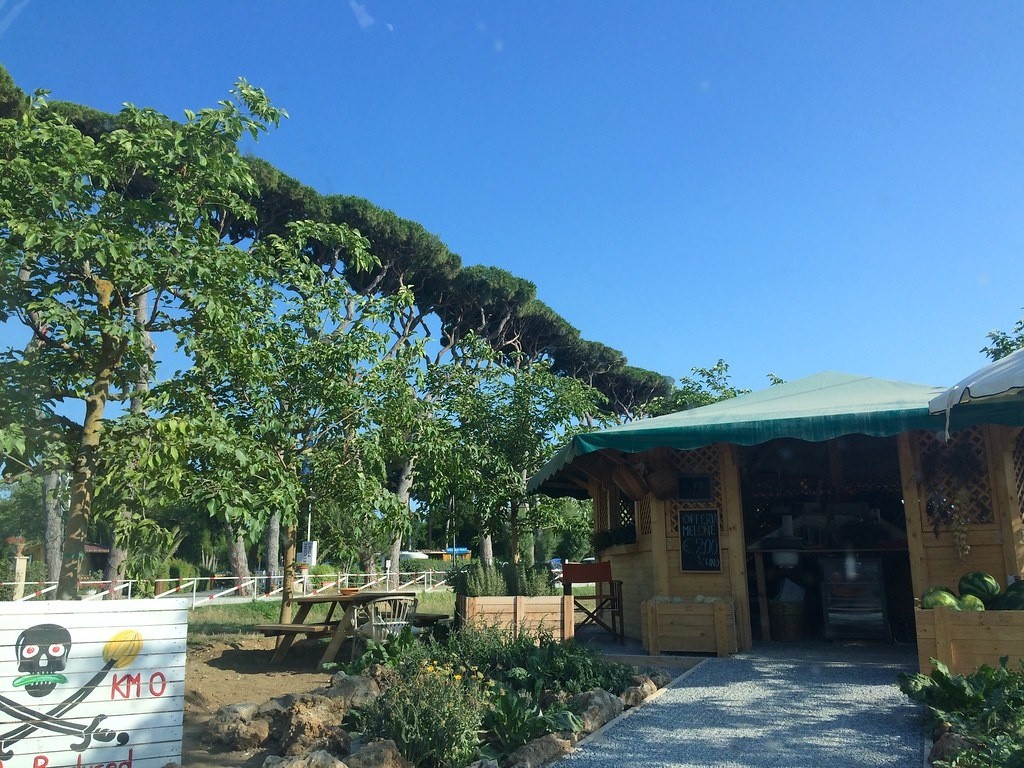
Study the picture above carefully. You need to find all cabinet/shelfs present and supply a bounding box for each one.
[820,554,890,640]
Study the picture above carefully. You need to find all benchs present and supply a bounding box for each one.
[254,624,337,640]
[359,612,449,627]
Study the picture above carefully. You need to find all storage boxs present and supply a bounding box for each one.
[914,598,1024,677]
[641,597,740,658]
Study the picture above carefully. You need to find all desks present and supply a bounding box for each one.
[269,593,416,673]
[747,514,909,640]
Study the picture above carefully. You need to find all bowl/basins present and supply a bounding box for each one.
[339,588,359,595]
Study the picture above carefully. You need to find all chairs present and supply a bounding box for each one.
[351,596,419,663]
[562,561,625,647]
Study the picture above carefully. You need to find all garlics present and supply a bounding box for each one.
[952,500,970,555]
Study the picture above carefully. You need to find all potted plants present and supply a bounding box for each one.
[444,555,564,647]
[82,569,103,595]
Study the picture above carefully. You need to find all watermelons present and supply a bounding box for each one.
[921,572,1024,612]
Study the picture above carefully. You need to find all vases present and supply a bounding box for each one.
[339,588,359,595]
